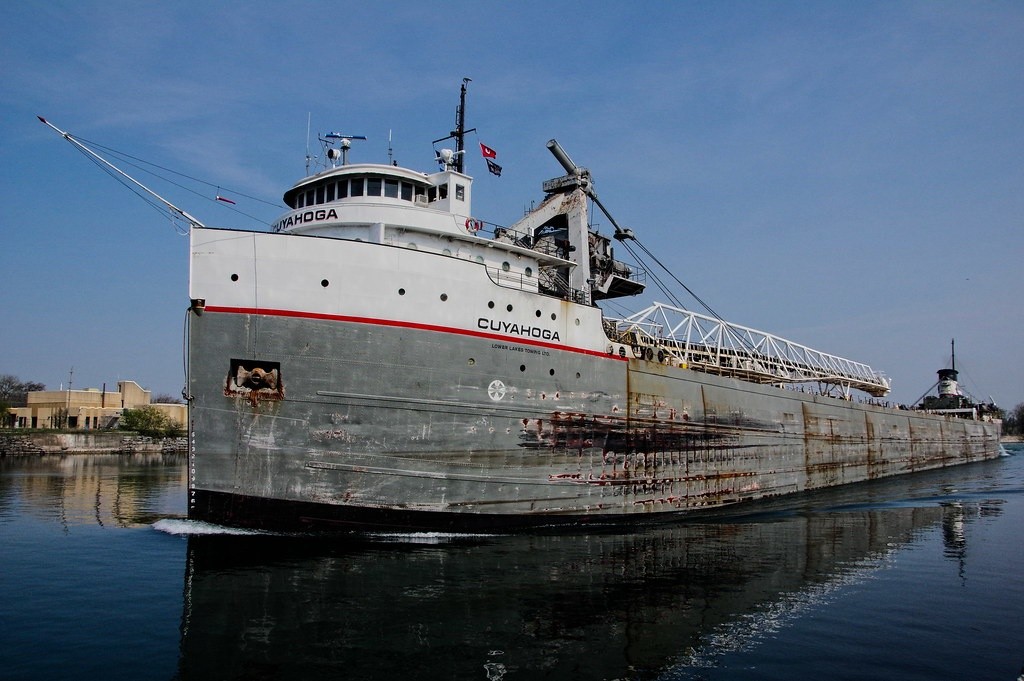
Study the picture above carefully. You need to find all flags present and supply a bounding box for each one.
[479,142,496,159]
[485,157,503,177]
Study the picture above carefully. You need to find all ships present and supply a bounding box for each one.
[38,77,1003,538]
